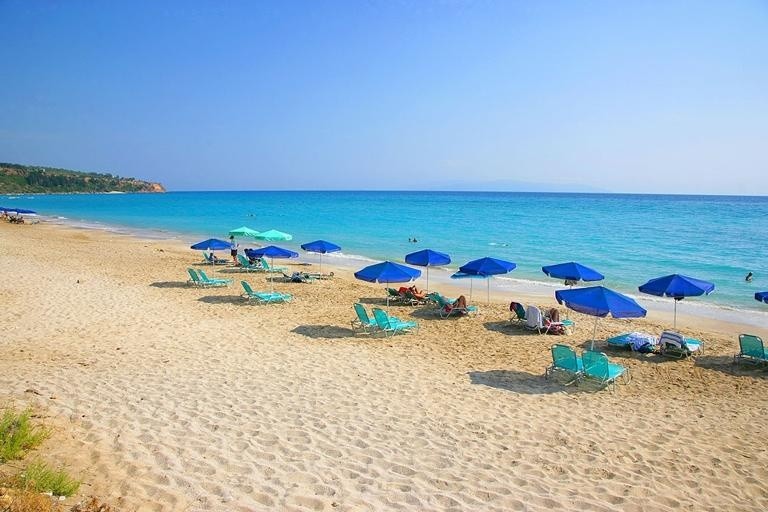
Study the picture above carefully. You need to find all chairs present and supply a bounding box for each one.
[196,268,232,289]
[426,292,441,312]
[239,280,292,307]
[434,292,477,319]
[526,304,575,336]
[580,347,629,394]
[372,307,419,339]
[203,251,287,273]
[510,302,528,329]
[734,332,768,369]
[404,289,431,307]
[545,343,584,387]
[185,268,202,287]
[659,329,705,358]
[282,272,317,284]
[351,302,399,336]
[384,287,404,305]
[606,330,655,353]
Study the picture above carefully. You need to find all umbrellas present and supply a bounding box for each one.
[191,237,233,268]
[245,244,300,292]
[9,208,36,216]
[680,297,684,298]
[254,228,294,244]
[0,206,16,213]
[457,254,517,302]
[352,259,422,320]
[449,270,496,303]
[753,290,768,304]
[552,284,649,352]
[226,225,260,237]
[637,272,716,331]
[403,248,453,296]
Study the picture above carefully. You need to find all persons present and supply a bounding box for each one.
[300,239,343,284]
[206,234,263,265]
[227,235,239,266]
[411,237,417,242]
[3,214,26,224]
[745,271,756,285]
[541,307,560,323]
[408,238,411,242]
[442,293,467,312]
[540,260,606,322]
[410,285,426,299]
